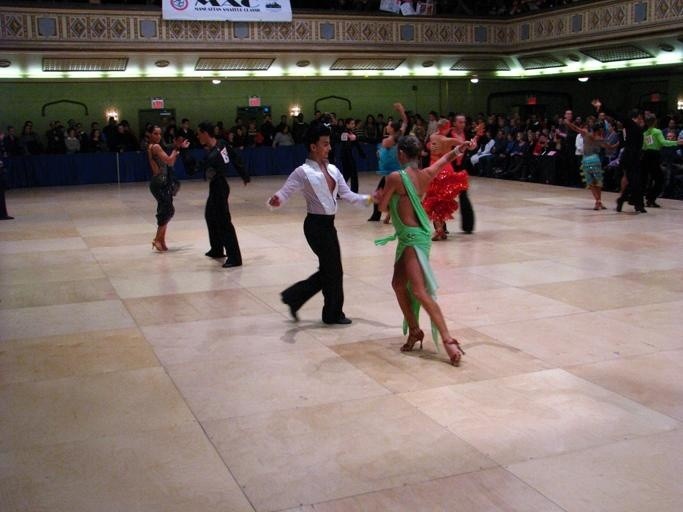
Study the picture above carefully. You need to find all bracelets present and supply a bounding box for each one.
[455,145,465,157]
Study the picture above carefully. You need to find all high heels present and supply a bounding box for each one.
[152,239,168,251]
[400,327,424,351]
[443,338,464,366]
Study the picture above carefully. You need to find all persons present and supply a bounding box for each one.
[173,121,251,269]
[375,134,478,367]
[156,110,363,199]
[0,114,141,158]
[145,121,190,251]
[477,100,682,213]
[365,103,487,240]
[1,134,14,220]
[266,125,379,327]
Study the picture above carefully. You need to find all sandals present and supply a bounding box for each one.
[593,200,607,210]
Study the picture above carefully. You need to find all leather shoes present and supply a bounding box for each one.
[223,263,232,266]
[0,216,15,220]
[282,298,297,320]
[616,194,660,212]
[205,251,211,256]
[339,317,352,324]
